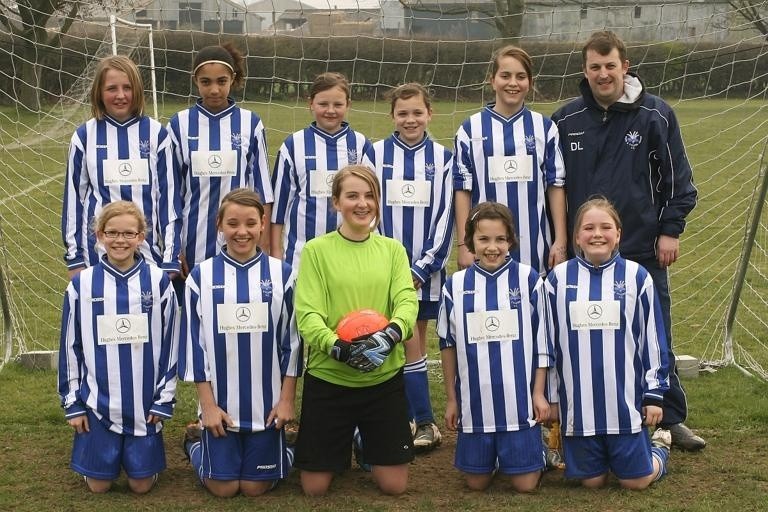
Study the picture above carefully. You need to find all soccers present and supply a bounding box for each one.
[338,309,390,343]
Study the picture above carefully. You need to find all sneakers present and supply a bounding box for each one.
[651,423,706,451]
[414,422,442,452]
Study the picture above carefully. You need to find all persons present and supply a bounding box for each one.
[56,200,181,495]
[453,45,568,281]
[360,82,455,450]
[545,30,707,450]
[61,54,183,281]
[435,201,562,492]
[177,187,305,499]
[292,164,420,497]
[544,198,672,490]
[271,71,372,274]
[165,41,275,281]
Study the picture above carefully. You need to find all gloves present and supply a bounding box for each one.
[334,324,402,371]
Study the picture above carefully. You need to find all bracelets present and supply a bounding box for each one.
[457,241,465,246]
